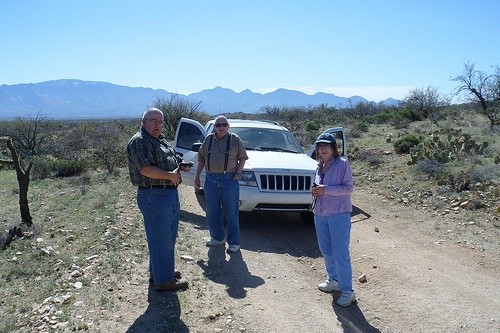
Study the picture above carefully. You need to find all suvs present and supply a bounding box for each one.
[174,117,347,225]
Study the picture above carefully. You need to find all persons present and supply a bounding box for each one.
[195,115,249,252]
[310,132,356,307]
[126,108,191,292]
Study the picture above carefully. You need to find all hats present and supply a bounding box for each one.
[314,133,336,144]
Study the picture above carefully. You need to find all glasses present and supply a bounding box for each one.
[214,122,229,128]
[142,118,165,125]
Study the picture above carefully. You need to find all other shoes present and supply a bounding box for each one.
[154,277,189,291]
[336,290,356,307]
[227,244,241,253]
[318,279,343,292]
[206,237,226,246]
[149,271,181,282]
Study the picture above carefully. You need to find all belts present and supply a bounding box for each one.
[138,185,178,189]
